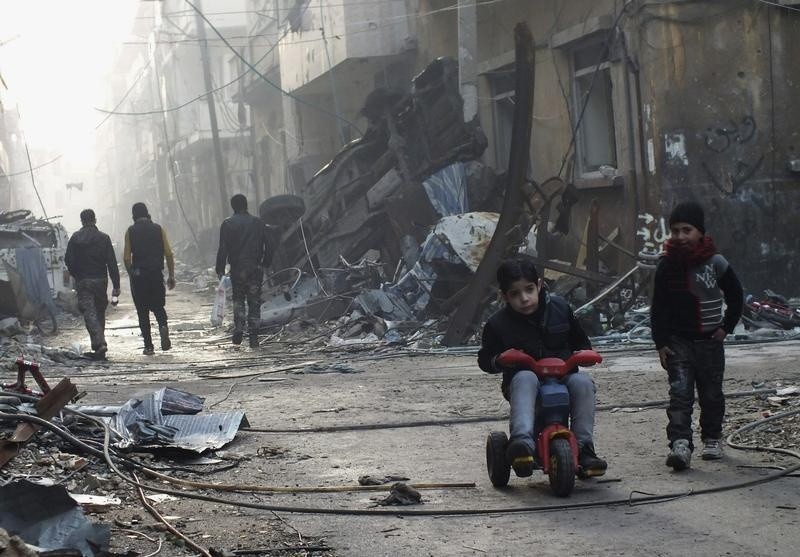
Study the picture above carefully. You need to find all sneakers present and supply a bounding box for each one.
[504,434,538,478]
[575,443,607,481]
[666,438,692,469]
[701,437,724,460]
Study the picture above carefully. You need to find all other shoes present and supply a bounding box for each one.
[232,331,243,345]
[94,346,107,359]
[161,339,172,350]
[143,348,155,355]
[250,341,259,349]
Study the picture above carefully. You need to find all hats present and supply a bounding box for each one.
[670,202,706,235]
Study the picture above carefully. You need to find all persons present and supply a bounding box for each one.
[216,193,272,347]
[124,202,176,355]
[64,209,121,352]
[651,203,743,469]
[478,259,608,477]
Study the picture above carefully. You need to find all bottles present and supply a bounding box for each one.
[112,289,118,302]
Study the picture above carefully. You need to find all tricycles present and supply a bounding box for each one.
[484,348,606,499]
